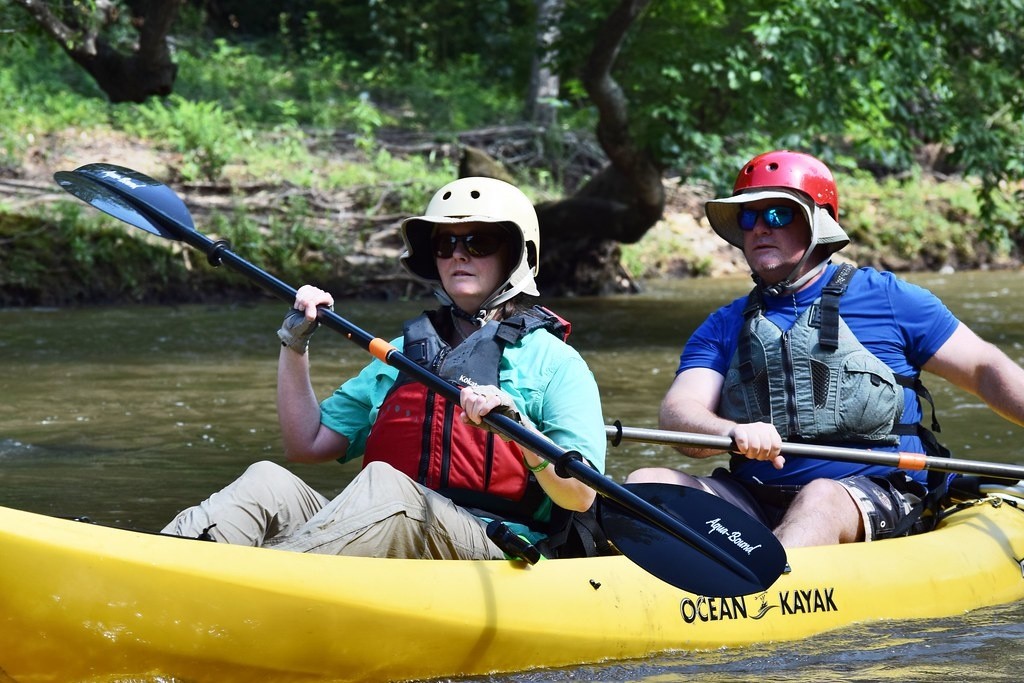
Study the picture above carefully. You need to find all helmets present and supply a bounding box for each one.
[733,151,838,223]
[425,177,539,277]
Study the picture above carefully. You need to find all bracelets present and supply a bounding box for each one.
[523,457,551,473]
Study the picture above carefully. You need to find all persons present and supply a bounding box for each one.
[624,152,1023,547]
[159,177,606,560]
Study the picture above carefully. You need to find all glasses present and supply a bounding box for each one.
[736,205,795,231]
[431,229,507,259]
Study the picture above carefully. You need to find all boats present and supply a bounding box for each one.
[0,477,1024,683]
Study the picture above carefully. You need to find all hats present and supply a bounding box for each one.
[704,187,851,257]
[399,216,541,297]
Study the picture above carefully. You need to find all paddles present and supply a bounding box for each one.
[51,162,787,599]
[602,420,1024,483]
